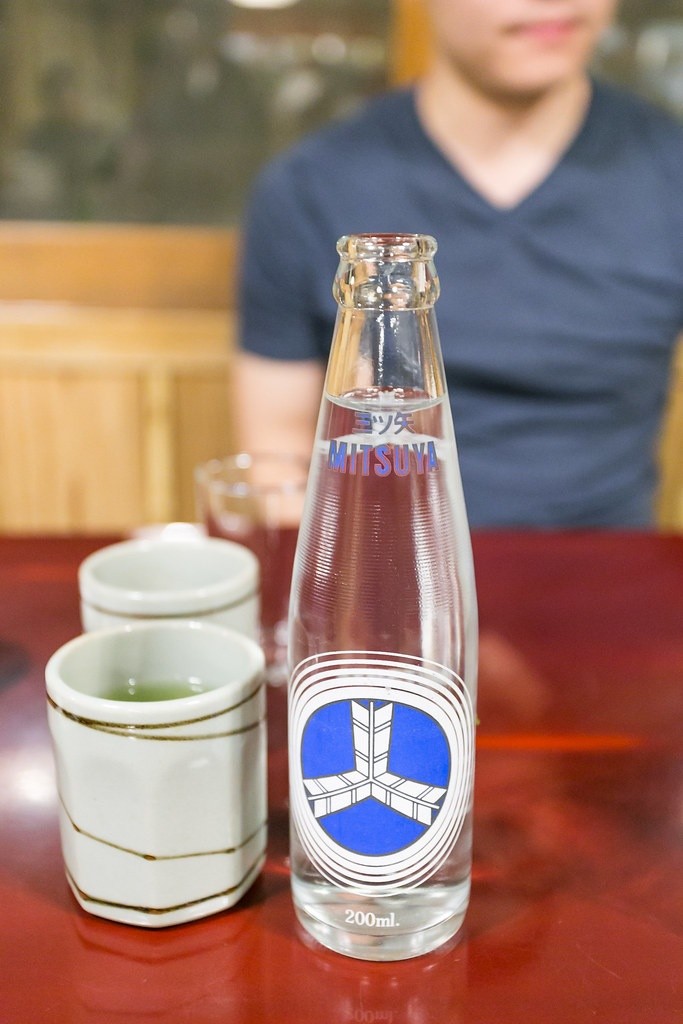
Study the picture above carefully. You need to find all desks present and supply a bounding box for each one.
[0,528,683,1024]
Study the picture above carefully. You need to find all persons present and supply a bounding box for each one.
[232,0,683,530]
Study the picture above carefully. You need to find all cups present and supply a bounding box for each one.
[45,618,271,929]
[78,521,264,646]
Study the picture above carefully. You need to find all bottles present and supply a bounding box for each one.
[286,231,478,964]
